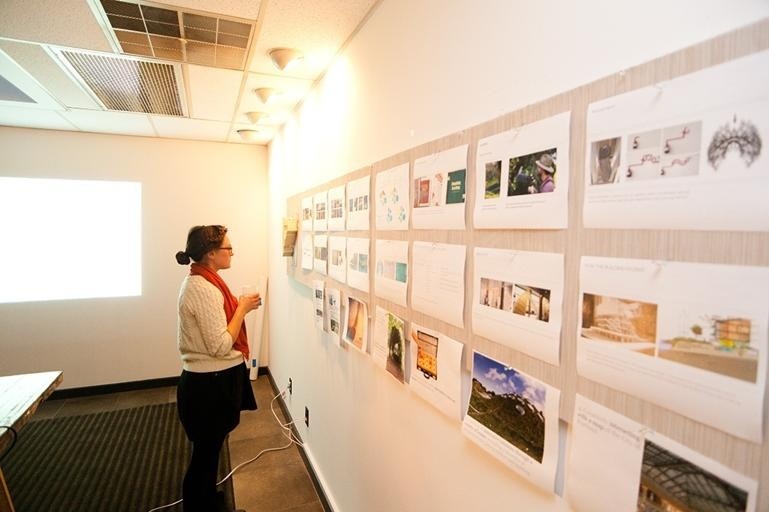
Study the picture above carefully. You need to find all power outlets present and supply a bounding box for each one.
[304,406,309,427]
[288,379,292,395]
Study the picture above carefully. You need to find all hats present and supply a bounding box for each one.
[535,154,554,173]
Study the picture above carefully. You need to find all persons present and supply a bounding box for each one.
[176,225,262,512]
[508,154,555,195]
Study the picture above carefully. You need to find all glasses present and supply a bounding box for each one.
[221,246,233,251]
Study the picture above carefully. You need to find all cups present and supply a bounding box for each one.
[243,285,259,298]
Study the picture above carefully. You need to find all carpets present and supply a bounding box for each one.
[0,402,235,512]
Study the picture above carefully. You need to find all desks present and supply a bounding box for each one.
[0,371,63,512]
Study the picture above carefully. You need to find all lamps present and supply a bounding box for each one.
[238,130,258,144]
[247,113,266,126]
[255,88,274,103]
[270,48,298,73]
